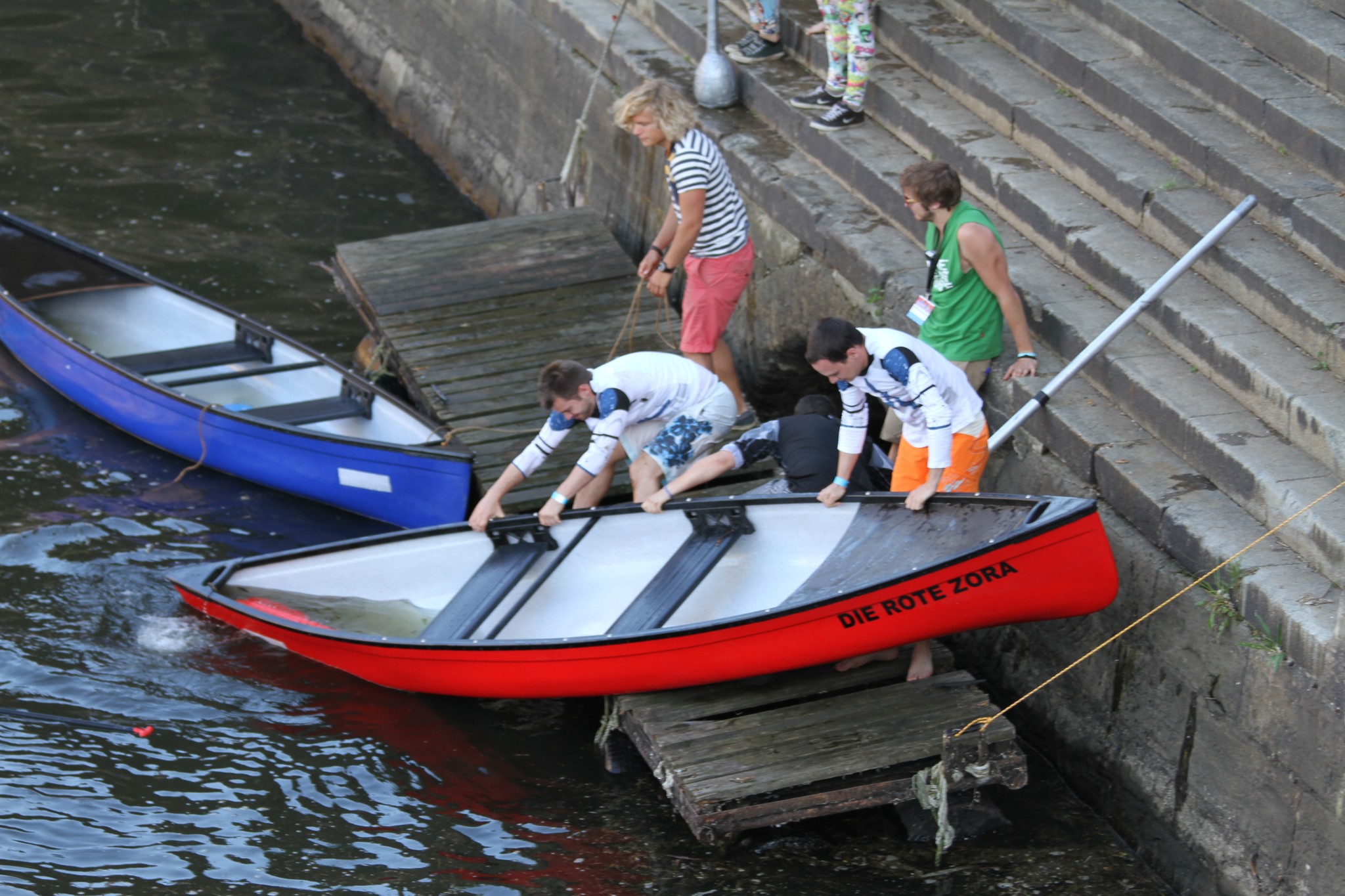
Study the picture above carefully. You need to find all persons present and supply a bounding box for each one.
[723,0,876,131]
[606,78,763,430]
[802,317,989,682]
[880,160,1039,465]
[466,350,738,533]
[637,394,892,515]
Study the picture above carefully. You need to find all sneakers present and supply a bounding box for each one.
[810,100,865,132]
[723,27,786,64]
[789,84,843,109]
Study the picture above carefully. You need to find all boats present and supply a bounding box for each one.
[163,490,1121,695]
[0,213,480,540]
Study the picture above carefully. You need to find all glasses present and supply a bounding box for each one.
[904,196,919,207]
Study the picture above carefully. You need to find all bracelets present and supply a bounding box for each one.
[832,476,851,489]
[1015,352,1038,361]
[662,485,674,501]
[551,490,569,505]
[649,244,665,258]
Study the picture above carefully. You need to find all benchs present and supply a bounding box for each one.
[107,321,376,426]
[416,503,756,643]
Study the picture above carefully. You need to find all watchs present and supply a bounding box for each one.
[657,260,675,273]
